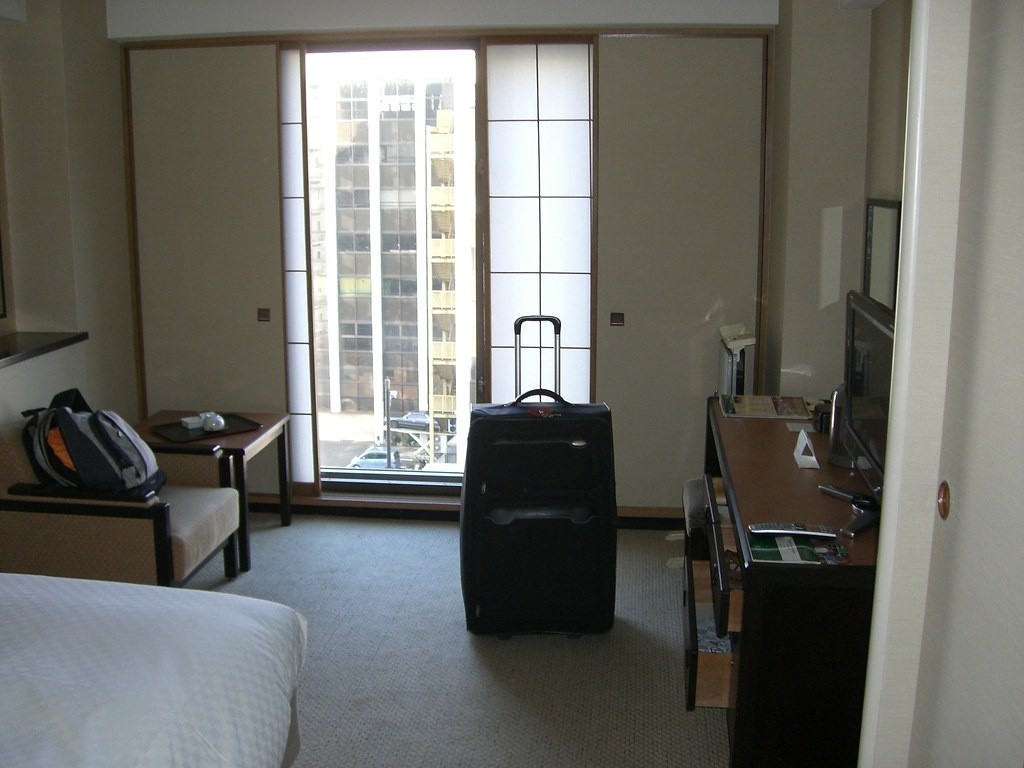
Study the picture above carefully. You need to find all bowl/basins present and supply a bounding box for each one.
[200,412,215,419]
[181,416,202,429]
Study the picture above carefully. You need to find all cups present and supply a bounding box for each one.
[203,414,225,430]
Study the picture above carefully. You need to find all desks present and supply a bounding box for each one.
[681,395,880,768]
[132,410,291,572]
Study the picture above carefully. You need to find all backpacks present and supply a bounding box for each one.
[21,408,167,502]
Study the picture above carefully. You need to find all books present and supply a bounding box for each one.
[719,394,814,419]
[748,534,821,564]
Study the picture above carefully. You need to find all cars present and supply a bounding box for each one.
[390,411,440,431]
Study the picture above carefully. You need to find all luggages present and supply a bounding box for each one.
[459,315,618,638]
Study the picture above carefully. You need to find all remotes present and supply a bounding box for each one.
[748,522,837,539]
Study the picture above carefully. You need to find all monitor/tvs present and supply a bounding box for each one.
[819,290,896,538]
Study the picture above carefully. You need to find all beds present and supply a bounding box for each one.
[1,573,310,768]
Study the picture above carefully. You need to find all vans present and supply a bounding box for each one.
[351,448,400,469]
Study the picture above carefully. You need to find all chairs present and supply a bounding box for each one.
[0,421,240,589]
